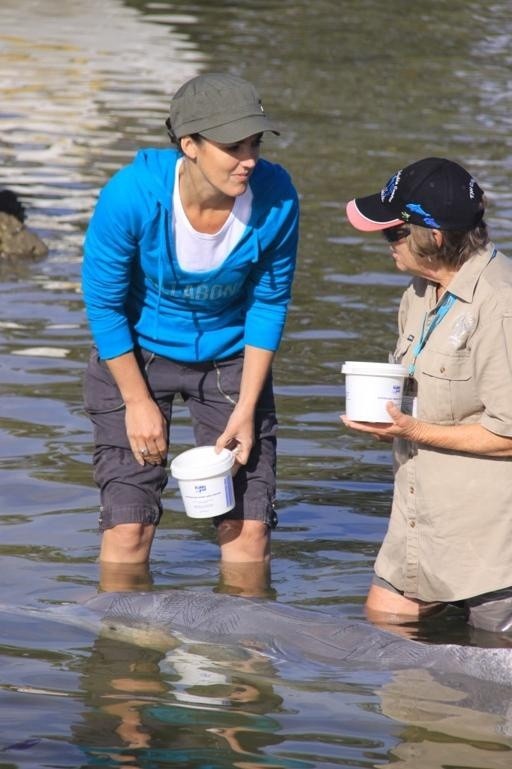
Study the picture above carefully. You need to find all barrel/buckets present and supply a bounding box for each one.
[340,360,409,423]
[169,446,237,518]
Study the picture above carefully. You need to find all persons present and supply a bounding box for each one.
[339,159,510,641]
[80,74,299,595]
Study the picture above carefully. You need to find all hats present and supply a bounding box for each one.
[345,157,484,232]
[165,72,280,145]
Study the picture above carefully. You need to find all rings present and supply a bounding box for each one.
[139,448,149,456]
